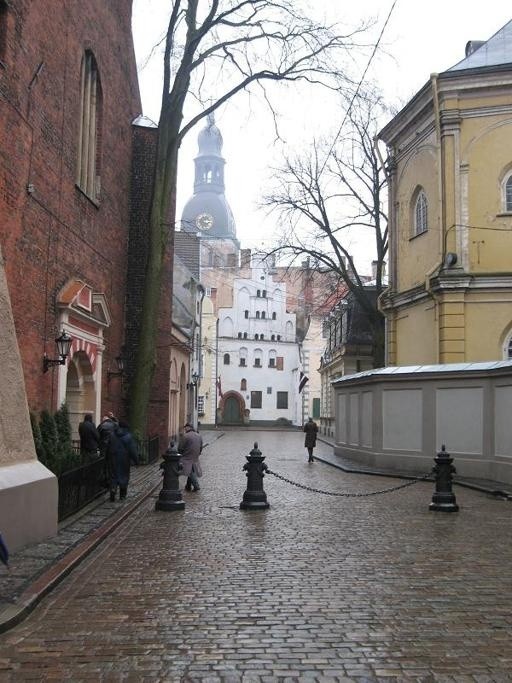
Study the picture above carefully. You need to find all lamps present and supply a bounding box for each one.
[42,328,74,374]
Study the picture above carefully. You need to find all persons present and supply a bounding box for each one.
[178,423,203,492]
[107,411,120,422]
[78,414,100,454]
[303,416,318,462]
[96,416,118,453]
[104,420,138,502]
